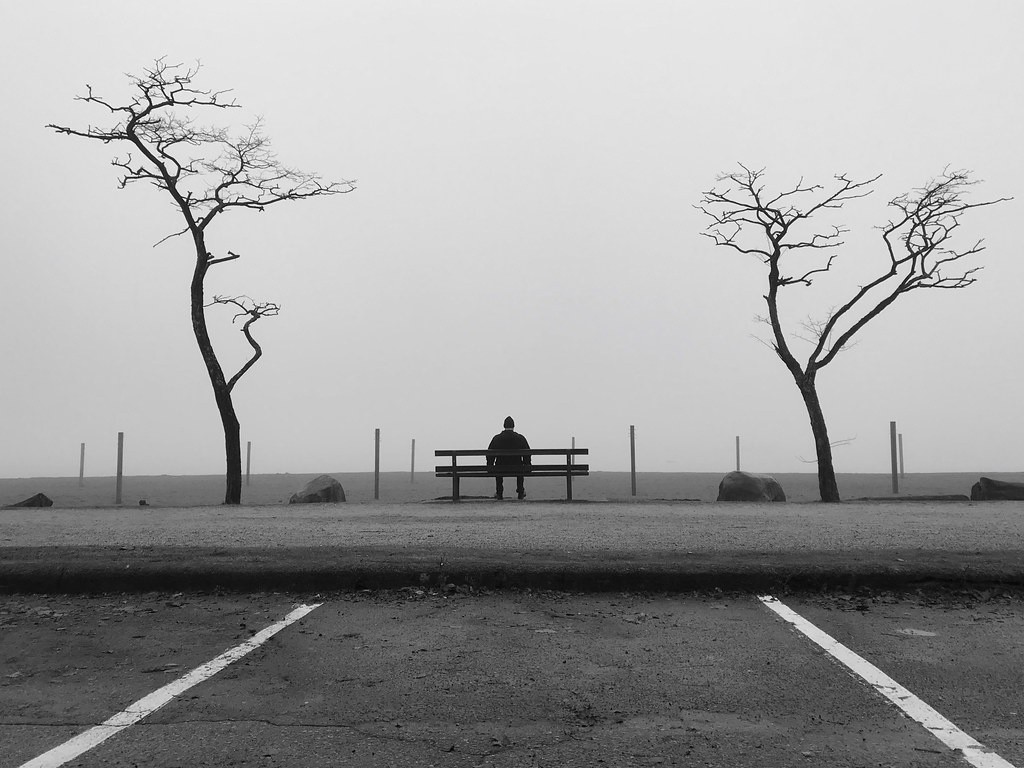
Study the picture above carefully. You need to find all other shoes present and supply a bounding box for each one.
[495,493,503,500]
[518,491,527,499]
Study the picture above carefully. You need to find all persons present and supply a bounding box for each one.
[486,416,531,499]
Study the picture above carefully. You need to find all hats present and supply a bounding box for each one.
[503,416,515,428]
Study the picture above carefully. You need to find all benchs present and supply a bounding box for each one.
[435,448,590,502]
